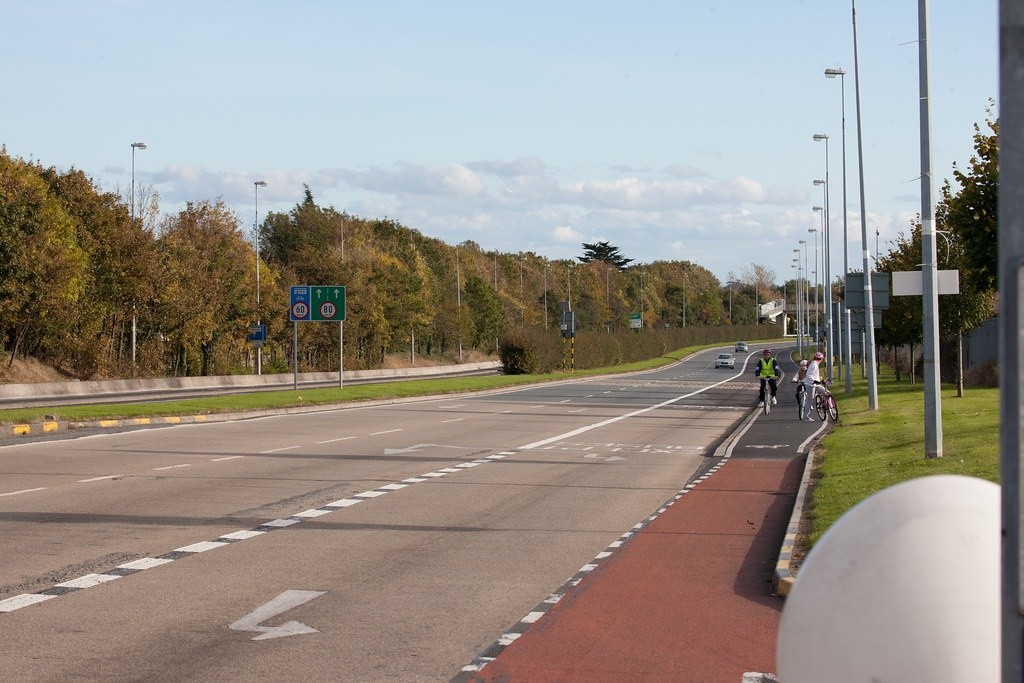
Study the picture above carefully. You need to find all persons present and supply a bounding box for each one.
[791,359,813,409]
[803,352,836,421]
[755,348,781,407]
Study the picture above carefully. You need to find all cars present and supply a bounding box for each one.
[714,353,736,369]
[734,342,749,353]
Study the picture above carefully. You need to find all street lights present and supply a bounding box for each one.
[456,244,464,362]
[130,142,149,379]
[812,133,835,380]
[606,267,613,320]
[493,252,501,353]
[790,227,821,359]
[340,212,350,261]
[824,67,854,393]
[567,264,573,301]
[640,272,646,327]
[520,257,528,329]
[253,179,269,375]
[543,263,550,333]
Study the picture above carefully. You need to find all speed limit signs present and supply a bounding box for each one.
[320,302,336,318]
[292,303,308,318]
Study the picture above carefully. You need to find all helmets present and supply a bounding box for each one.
[763,350,771,354]
[814,352,824,359]
[800,360,808,365]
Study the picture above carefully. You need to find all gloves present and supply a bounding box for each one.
[814,380,820,385]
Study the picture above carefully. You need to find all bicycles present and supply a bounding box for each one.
[811,377,839,421]
[754,376,778,415]
[790,380,808,421]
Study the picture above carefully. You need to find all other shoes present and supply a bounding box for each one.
[803,416,815,422]
[828,406,836,414]
[771,396,777,405]
[758,401,764,408]
